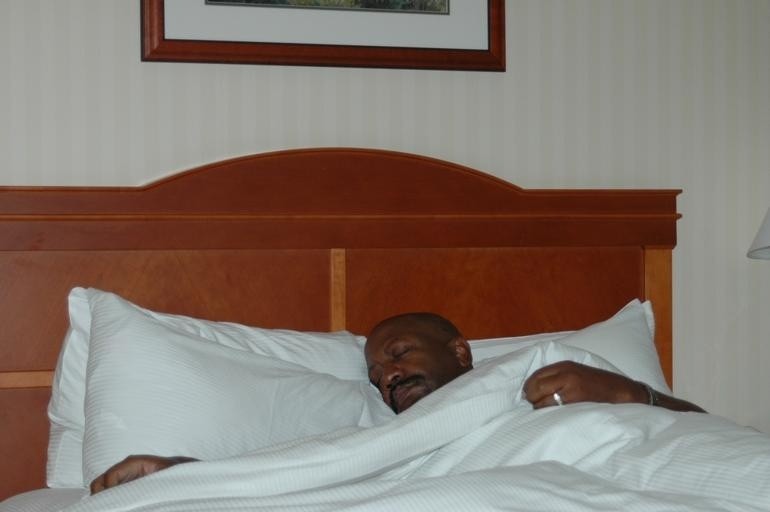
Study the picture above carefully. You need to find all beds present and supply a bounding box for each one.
[0,147,770,512]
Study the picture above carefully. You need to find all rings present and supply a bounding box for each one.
[552,392,564,405]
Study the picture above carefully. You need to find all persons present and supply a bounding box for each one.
[89,309,712,498]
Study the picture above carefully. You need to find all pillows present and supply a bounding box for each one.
[45,287,673,487]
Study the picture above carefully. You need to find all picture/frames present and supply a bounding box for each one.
[140,0,505,73]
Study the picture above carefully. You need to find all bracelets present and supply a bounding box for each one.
[638,382,659,406]
